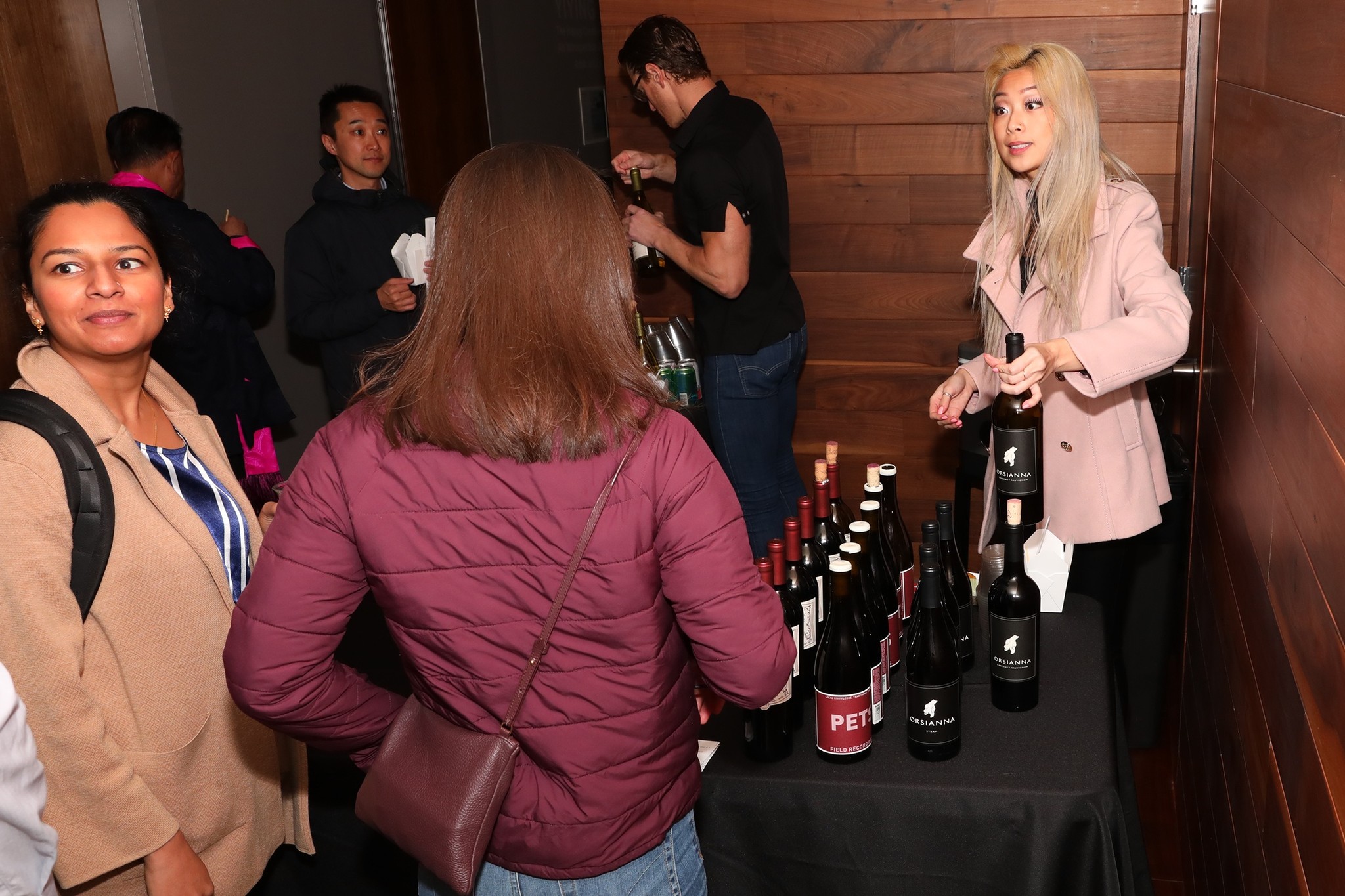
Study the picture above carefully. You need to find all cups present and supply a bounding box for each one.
[974,543,1004,682]
[642,312,696,366]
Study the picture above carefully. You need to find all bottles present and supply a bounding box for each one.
[630,168,668,276]
[985,498,1041,713]
[634,312,660,376]
[992,333,1045,528]
[755,440,975,766]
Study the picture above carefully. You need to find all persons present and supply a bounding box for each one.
[0,180,315,896]
[107,107,299,489]
[612,13,808,559]
[284,83,436,421]
[223,139,797,896]
[930,40,1194,736]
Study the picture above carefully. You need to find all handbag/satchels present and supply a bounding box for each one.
[353,694,520,896]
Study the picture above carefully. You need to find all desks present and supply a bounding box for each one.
[684,592,1156,896]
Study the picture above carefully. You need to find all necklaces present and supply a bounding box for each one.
[141,387,158,446]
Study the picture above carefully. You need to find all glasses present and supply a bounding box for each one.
[629,62,662,103]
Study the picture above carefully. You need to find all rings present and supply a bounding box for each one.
[1022,370,1027,381]
[1003,357,1006,360]
[943,393,951,398]
